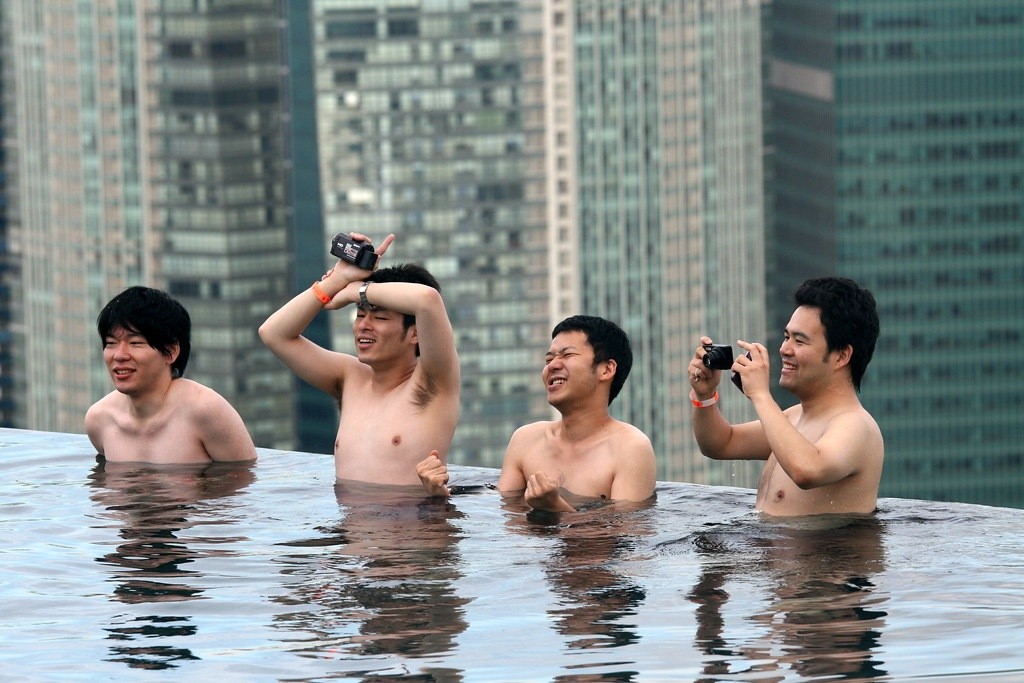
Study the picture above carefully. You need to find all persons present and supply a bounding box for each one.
[84,286,257,464]
[416,315,657,512]
[259,232,460,486]
[688,277,884,517]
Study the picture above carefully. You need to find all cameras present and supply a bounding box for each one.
[329,233,379,271]
[702,345,734,370]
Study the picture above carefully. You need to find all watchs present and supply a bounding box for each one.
[359,281,377,309]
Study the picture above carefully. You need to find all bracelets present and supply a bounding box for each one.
[312,282,330,303]
[690,389,718,407]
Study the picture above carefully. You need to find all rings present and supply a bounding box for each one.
[695,368,699,376]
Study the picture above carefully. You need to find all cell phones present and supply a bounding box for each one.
[731,352,752,394]
